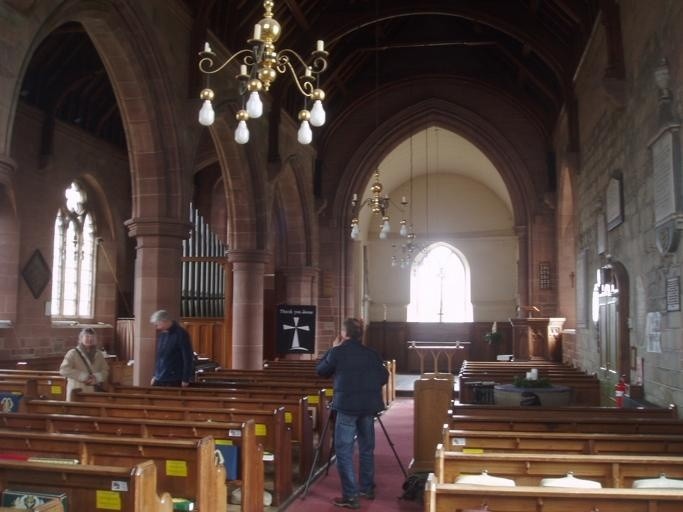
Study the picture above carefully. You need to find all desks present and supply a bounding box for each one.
[407,346,464,383]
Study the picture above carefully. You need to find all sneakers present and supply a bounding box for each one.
[332,495,360,509]
[357,489,375,501]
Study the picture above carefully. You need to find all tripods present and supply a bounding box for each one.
[300,407,407,501]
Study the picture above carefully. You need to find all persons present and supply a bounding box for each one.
[317,316,391,509]
[148,310,194,387]
[59,327,108,402]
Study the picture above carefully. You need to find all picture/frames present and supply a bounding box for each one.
[630,347,636,370]
[22,249,51,299]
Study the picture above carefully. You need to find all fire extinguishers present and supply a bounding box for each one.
[614,372,629,406]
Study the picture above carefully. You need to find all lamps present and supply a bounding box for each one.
[197,0,330,145]
[389,24,432,269]
[349,123,409,241]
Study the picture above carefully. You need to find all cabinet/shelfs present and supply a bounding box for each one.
[508,317,567,362]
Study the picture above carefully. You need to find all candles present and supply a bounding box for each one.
[412,341,415,347]
[456,341,459,349]
